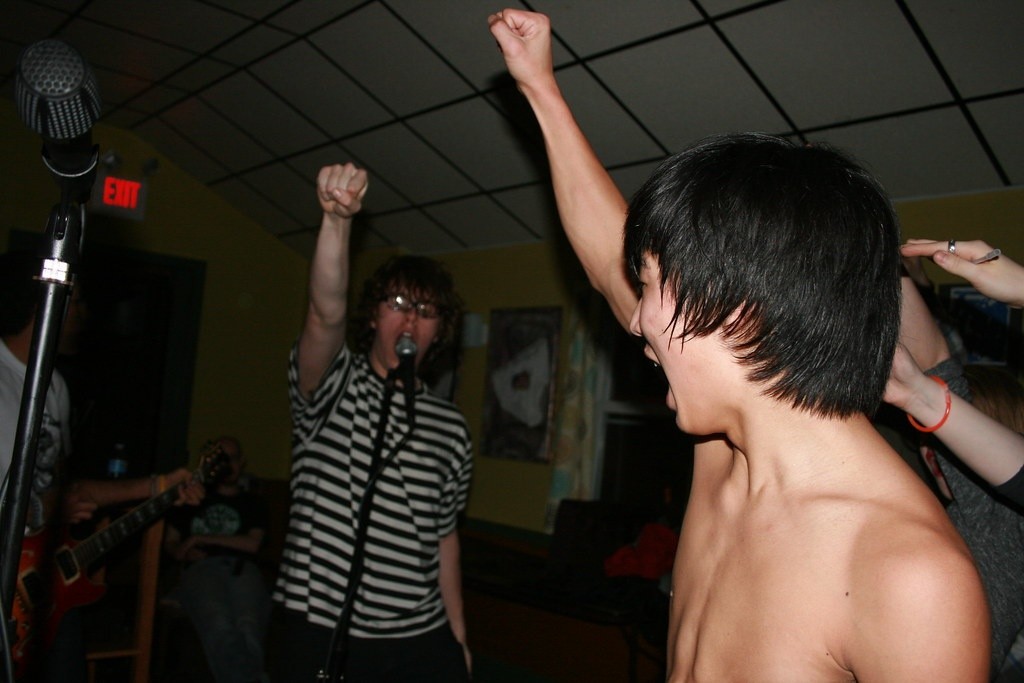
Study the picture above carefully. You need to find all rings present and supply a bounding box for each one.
[949,240,955,254]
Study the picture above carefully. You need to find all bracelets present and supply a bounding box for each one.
[160,475,167,494]
[906,375,951,432]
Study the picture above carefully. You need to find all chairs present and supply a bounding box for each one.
[83,471,170,682]
[541,497,667,683]
[152,477,294,683]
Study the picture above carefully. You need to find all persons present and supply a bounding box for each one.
[486,9,987,682]
[0,248,204,683]
[881,240,1023,683]
[166,435,270,683]
[273,162,474,683]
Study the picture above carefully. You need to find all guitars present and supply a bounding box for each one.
[1,440,230,668]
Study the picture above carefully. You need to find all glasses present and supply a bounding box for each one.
[381,293,440,319]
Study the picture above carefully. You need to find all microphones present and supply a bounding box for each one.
[12,38,100,194]
[395,339,418,426]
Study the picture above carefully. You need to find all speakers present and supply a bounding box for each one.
[546,498,646,623]
